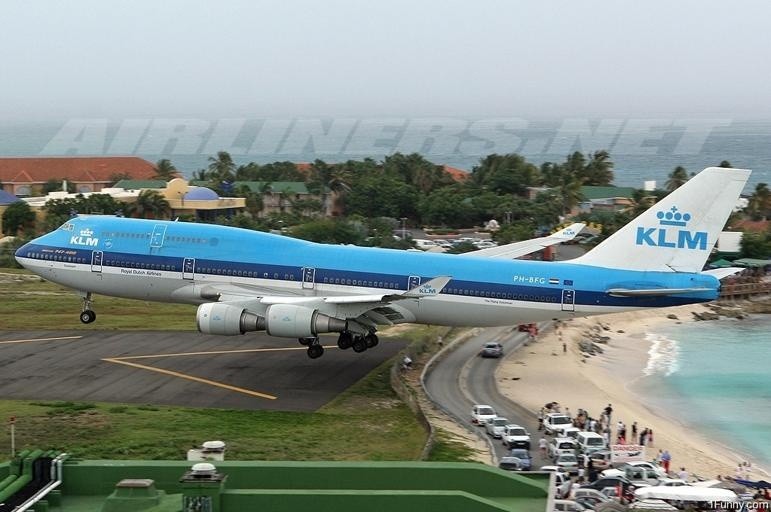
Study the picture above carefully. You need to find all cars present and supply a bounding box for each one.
[467,401,753,512]
[477,339,505,359]
[364,228,500,254]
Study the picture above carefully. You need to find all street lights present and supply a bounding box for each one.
[277,216,289,235]
[504,210,512,225]
[401,217,409,239]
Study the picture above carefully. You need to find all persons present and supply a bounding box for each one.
[539,397,771,511]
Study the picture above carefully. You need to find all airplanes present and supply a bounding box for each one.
[11,164,753,363]
